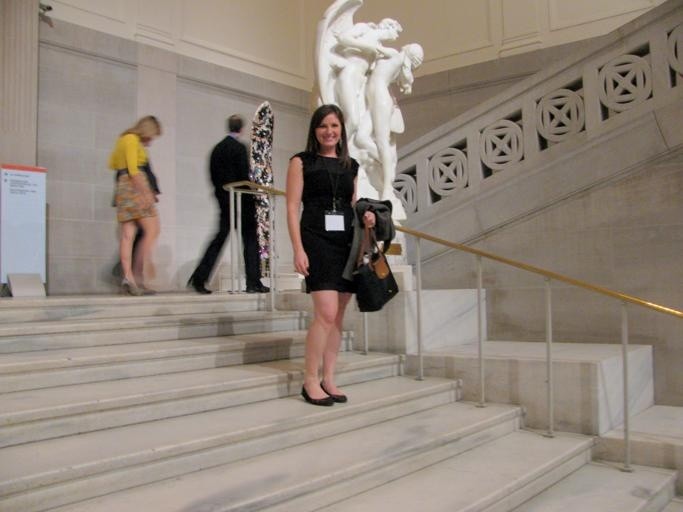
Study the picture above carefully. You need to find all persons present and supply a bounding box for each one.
[185,112,270,296]
[105,115,164,297]
[334,17,424,207]
[284,103,378,407]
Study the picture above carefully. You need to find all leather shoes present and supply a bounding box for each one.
[246,282,270,292]
[320,382,347,403]
[301,385,334,406]
[188,275,212,294]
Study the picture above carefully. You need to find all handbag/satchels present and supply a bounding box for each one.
[351,252,399,312]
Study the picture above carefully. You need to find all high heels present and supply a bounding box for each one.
[120,279,157,295]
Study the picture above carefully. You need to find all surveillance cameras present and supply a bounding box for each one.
[39,3,53,14]
